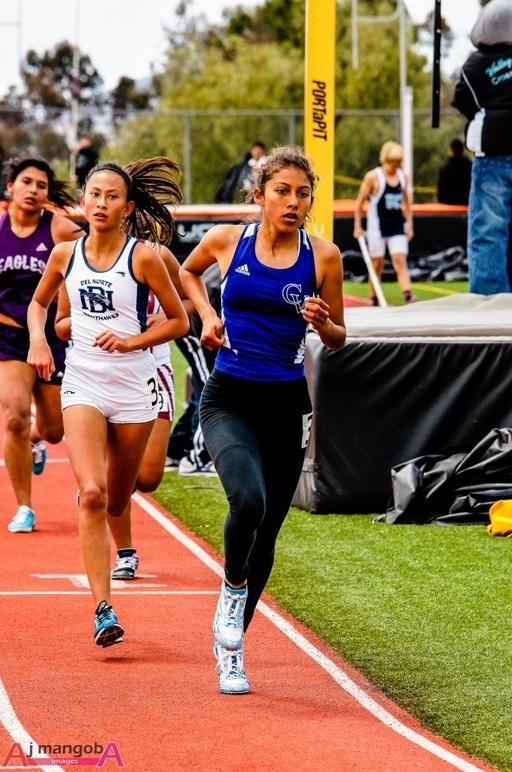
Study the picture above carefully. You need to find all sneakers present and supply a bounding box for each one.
[211,579,250,652]
[29,441,48,476]
[212,641,251,696]
[92,600,126,649]
[6,505,36,534]
[164,456,219,477]
[111,547,140,581]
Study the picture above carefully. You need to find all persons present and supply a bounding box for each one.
[0,0,512,696]
